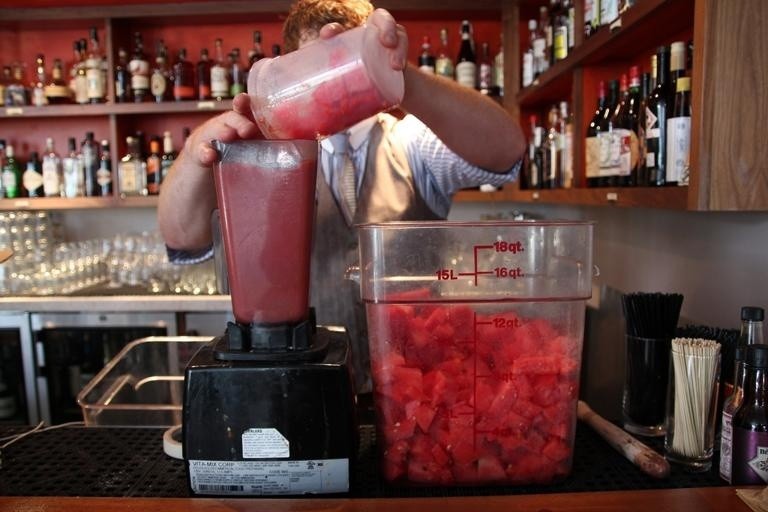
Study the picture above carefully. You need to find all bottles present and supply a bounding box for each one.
[415,20,505,99]
[3,22,109,107]
[520,1,632,90]
[0,128,112,198]
[112,29,280,103]
[518,99,576,193]
[584,39,694,189]
[716,305,768,487]
[111,125,192,198]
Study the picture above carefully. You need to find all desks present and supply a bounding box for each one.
[2,422,768,512]
[1,283,242,430]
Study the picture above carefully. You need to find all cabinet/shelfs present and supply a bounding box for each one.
[1,2,521,211]
[514,0,768,223]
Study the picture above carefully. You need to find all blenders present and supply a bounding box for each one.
[155,135,359,495]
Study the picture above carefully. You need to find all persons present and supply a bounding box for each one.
[156,0,527,425]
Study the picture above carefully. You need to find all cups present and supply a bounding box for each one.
[93,226,221,299]
[663,349,722,464]
[618,330,674,438]
[246,25,406,142]
[360,219,595,485]
[0,208,115,298]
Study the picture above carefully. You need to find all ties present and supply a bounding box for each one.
[329,132,360,229]
